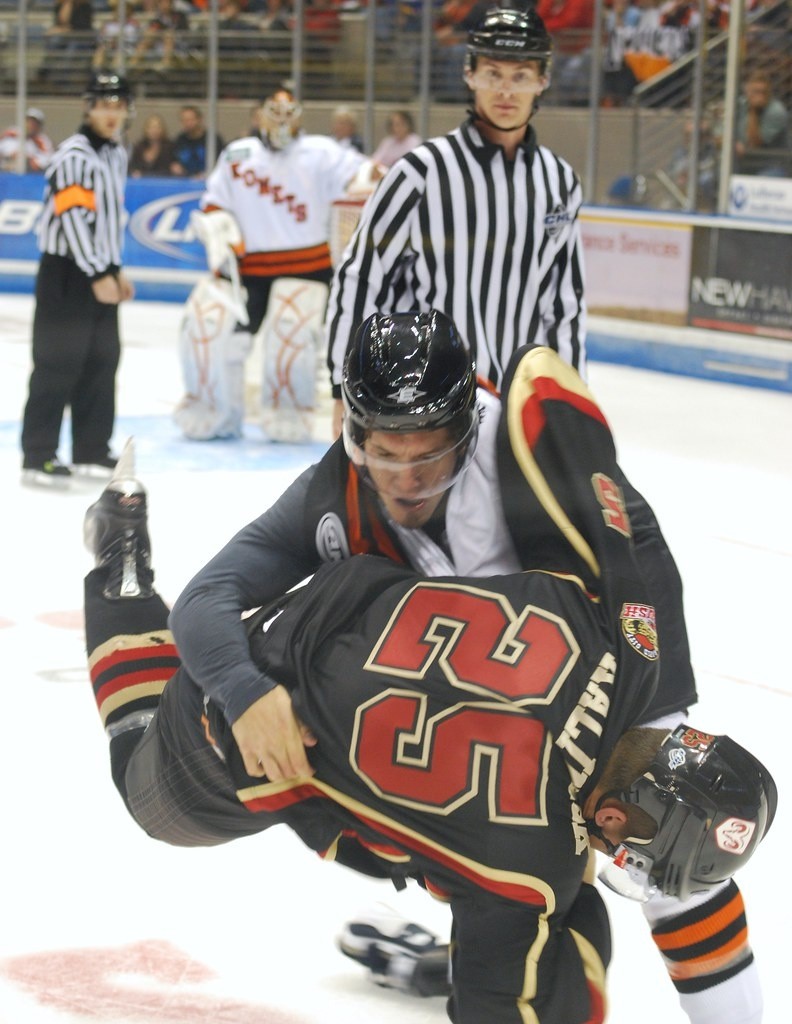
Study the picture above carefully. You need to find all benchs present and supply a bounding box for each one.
[0,0,792,215]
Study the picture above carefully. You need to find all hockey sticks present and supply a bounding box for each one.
[197,247,250,326]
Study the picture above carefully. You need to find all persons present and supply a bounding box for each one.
[321,5,590,443]
[22,73,139,487]
[84,307,779,1024]
[175,87,389,443]
[0,0,787,180]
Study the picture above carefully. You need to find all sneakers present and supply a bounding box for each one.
[21,454,72,487]
[74,453,118,479]
[83,436,154,601]
[338,918,439,999]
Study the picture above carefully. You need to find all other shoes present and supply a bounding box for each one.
[265,419,316,443]
[181,415,245,440]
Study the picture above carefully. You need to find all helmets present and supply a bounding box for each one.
[597,723,778,904]
[259,88,303,148]
[341,310,480,501]
[81,72,136,118]
[463,8,553,92]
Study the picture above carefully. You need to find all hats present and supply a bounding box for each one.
[27,107,46,124]
